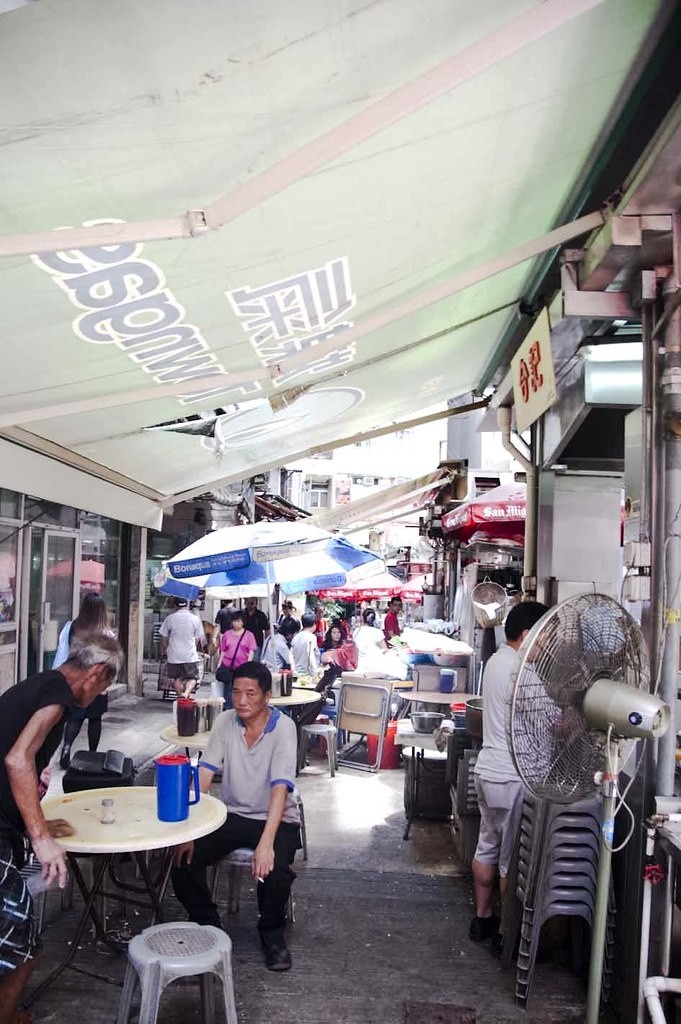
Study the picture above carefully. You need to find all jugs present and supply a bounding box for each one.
[152,754,199,822]
[177,700,197,736]
[279,669,292,696]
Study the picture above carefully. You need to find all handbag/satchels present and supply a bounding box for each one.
[216,666,233,683]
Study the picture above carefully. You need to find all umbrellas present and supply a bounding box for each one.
[155,519,387,672]
[308,572,447,622]
[48,558,105,586]
[439,476,527,541]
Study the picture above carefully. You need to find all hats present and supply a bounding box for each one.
[176,597,187,606]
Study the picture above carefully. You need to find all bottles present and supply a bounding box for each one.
[100,799,115,825]
[421,576,430,594]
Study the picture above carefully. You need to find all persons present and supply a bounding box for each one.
[212,596,423,710]
[469,601,585,953]
[171,661,301,971]
[159,598,204,699]
[0,626,126,1024]
[52,592,117,768]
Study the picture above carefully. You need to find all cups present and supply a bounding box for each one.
[196,706,206,733]
[440,669,457,692]
[272,680,281,699]
[208,706,222,731]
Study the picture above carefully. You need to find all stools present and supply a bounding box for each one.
[209,846,295,924]
[293,789,308,862]
[499,785,617,1012]
[298,723,339,778]
[115,922,237,1024]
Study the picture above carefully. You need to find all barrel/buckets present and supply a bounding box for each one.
[471,582,507,629]
[465,699,483,738]
[368,721,400,769]
[402,747,451,821]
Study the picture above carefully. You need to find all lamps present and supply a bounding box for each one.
[575,335,643,362]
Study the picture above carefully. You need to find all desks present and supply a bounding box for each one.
[22,786,227,1009]
[398,691,483,714]
[268,688,322,778]
[334,674,393,773]
[394,718,456,840]
[159,723,212,768]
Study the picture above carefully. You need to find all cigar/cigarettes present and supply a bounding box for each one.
[257,876,265,883]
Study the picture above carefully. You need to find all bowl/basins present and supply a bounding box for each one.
[450,712,465,727]
[408,712,446,733]
[427,655,434,663]
[409,653,426,663]
[433,653,467,667]
[450,703,466,711]
[427,585,442,595]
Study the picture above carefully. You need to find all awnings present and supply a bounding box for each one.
[295,469,456,535]
[281,469,304,481]
[0,1,681,529]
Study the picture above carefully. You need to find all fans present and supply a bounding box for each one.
[505,593,671,1024]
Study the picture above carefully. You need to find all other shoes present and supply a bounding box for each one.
[494,942,553,963]
[60,745,71,768]
[262,938,291,970]
[469,914,501,940]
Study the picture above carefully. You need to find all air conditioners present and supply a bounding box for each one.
[361,477,374,486]
